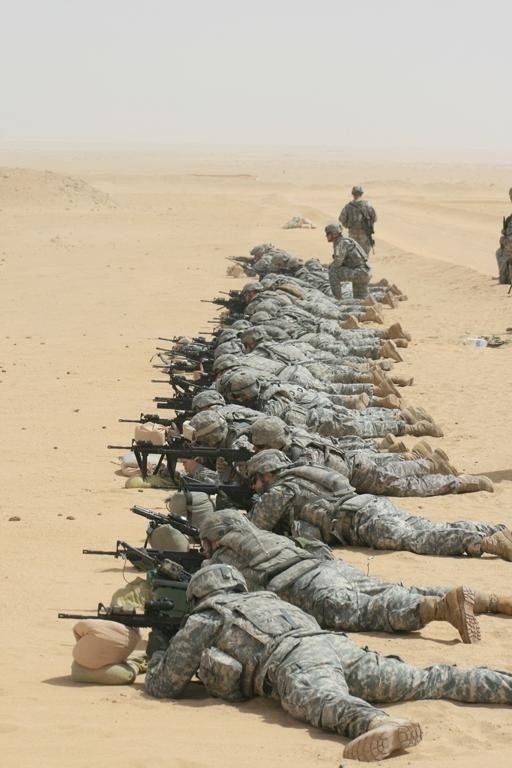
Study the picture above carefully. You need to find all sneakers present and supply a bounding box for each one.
[497,595,512,615]
[482,525,512,562]
[418,584,481,643]
[343,278,495,494]
[343,713,424,762]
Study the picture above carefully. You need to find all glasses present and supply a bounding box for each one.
[326,231,331,236]
[250,475,256,486]
[197,547,205,554]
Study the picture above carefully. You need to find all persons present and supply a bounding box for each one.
[323,223,394,307]
[143,241,511,762]
[496,189,511,286]
[339,185,377,255]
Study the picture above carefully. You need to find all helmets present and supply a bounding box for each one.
[325,224,343,239]
[248,415,291,450]
[199,509,244,542]
[246,449,293,478]
[190,273,281,448]
[351,185,363,195]
[251,244,320,271]
[184,564,249,602]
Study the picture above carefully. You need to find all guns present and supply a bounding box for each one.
[199,287,248,328]
[82,549,206,573]
[117,414,188,434]
[228,249,259,275]
[130,503,205,552]
[172,474,257,514]
[149,330,219,414]
[358,208,375,252]
[106,438,250,477]
[58,597,188,646]
[499,216,508,239]
